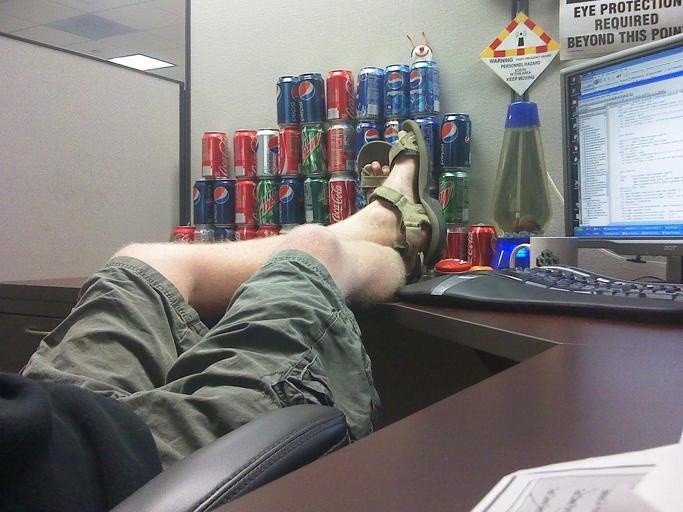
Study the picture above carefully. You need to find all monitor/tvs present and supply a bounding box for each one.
[561,32,683,279]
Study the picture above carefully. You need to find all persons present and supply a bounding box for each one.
[0,119,447,512]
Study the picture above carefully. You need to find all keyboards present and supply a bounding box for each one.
[393,262,682,320]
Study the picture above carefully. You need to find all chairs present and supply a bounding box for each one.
[110,404,349,511]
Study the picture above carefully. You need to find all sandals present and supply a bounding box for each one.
[369,119,447,266]
[357,140,425,285]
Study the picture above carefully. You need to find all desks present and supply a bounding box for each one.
[0,277,683,512]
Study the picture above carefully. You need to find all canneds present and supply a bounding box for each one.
[233,130,257,179]
[235,226,256,242]
[354,176,367,211]
[173,225,194,243]
[443,223,466,262]
[275,75,298,126]
[354,118,382,178]
[277,175,304,225]
[211,177,235,228]
[193,227,215,242]
[253,177,280,226]
[234,177,257,229]
[383,63,409,118]
[328,174,354,226]
[214,225,237,242]
[437,172,471,226]
[326,69,356,121]
[201,131,230,179]
[301,124,328,179]
[466,223,496,267]
[427,172,438,200]
[356,66,384,119]
[298,72,326,126]
[409,60,440,117]
[254,226,281,240]
[193,179,212,227]
[328,122,355,176]
[257,127,280,180]
[303,175,328,225]
[382,115,407,144]
[279,126,302,176]
[411,114,439,170]
[439,112,472,171]
